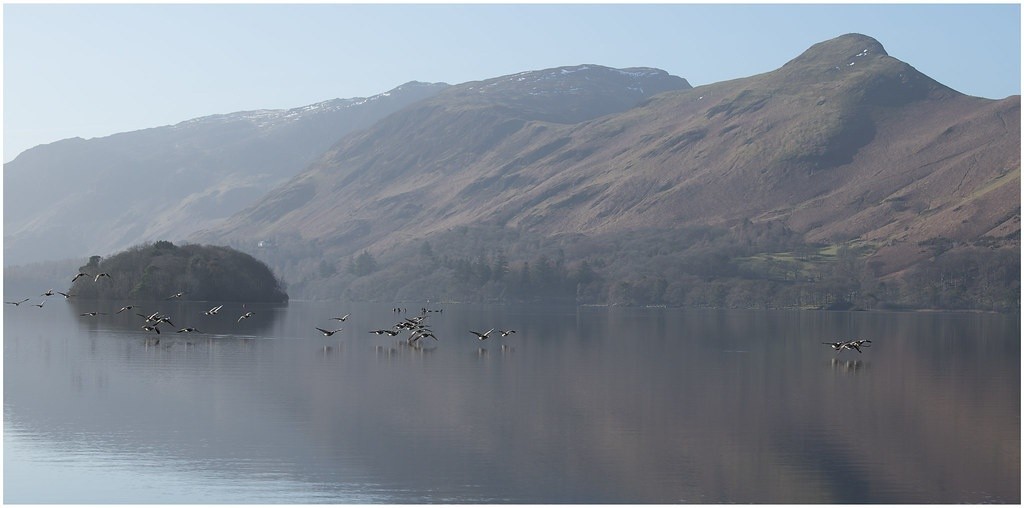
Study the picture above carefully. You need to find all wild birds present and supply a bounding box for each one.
[79,312,98,317]
[203,305,224,316]
[141,325,161,335]
[115,305,136,314]
[498,330,516,337]
[169,293,182,299]
[71,273,90,282]
[176,328,202,335]
[830,340,871,354]
[315,327,344,336]
[237,311,255,323]
[469,328,495,341]
[137,311,174,327]
[40,289,54,296]
[4,298,29,306]
[35,300,45,308]
[332,312,351,322]
[57,292,75,298]
[369,330,385,335]
[94,273,111,282]
[385,308,443,342]
